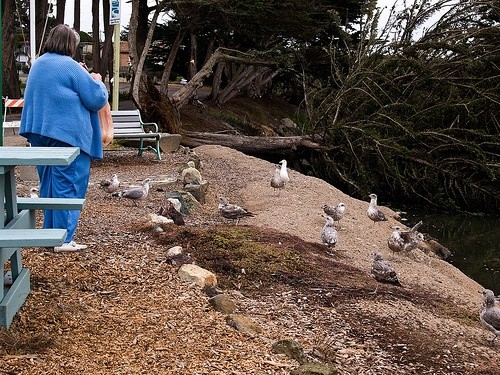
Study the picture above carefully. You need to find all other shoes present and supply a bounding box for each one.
[54,241,88,252]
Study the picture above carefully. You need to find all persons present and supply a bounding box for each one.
[18,24,108,253]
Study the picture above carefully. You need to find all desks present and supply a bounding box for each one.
[0,147,81,329]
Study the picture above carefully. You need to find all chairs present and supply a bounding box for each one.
[2,96,24,141]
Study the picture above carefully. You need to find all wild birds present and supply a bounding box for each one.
[369,252,404,295]
[270,159,290,197]
[388,221,426,259]
[218,195,259,228]
[321,203,346,252]
[367,193,389,228]
[101,174,154,208]
[477,288,500,341]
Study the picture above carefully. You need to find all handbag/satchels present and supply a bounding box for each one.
[99,100,113,147]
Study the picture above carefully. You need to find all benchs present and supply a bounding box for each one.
[0,229,68,247]
[111,110,162,162]
[3,196,86,210]
